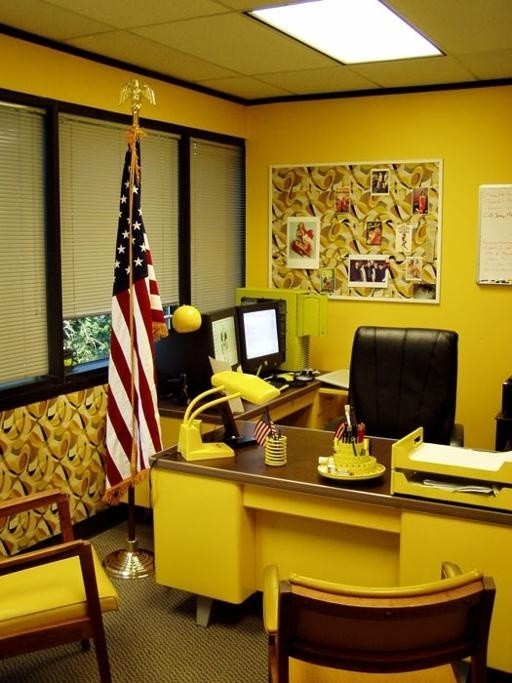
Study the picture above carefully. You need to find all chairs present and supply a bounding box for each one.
[347,326,459,447]
[258,562,496,682]
[0,483,119,682]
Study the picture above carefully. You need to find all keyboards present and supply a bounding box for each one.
[264,378,290,391]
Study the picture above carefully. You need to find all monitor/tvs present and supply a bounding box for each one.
[169,302,287,372]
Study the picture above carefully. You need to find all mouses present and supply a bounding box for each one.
[274,373,294,381]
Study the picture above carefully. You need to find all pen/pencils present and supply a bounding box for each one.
[268,430,282,461]
[336,401,366,456]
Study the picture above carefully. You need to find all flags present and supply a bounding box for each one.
[102,141,169,506]
[253,414,277,446]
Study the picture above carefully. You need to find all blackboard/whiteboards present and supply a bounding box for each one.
[475,184,512,285]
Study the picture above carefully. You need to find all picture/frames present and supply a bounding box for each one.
[266,158,442,305]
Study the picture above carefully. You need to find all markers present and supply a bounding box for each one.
[480,279,509,283]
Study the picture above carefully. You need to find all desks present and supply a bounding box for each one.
[113,373,330,512]
[145,414,512,677]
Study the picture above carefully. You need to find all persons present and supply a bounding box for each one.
[351,260,385,282]
[292,222,313,258]
[418,191,426,213]
